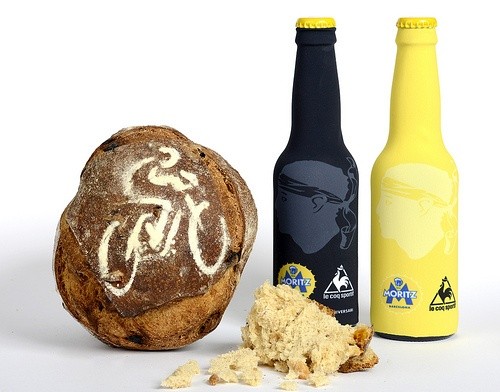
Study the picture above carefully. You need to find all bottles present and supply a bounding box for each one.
[369,17,462,339]
[271,17,361,327]
